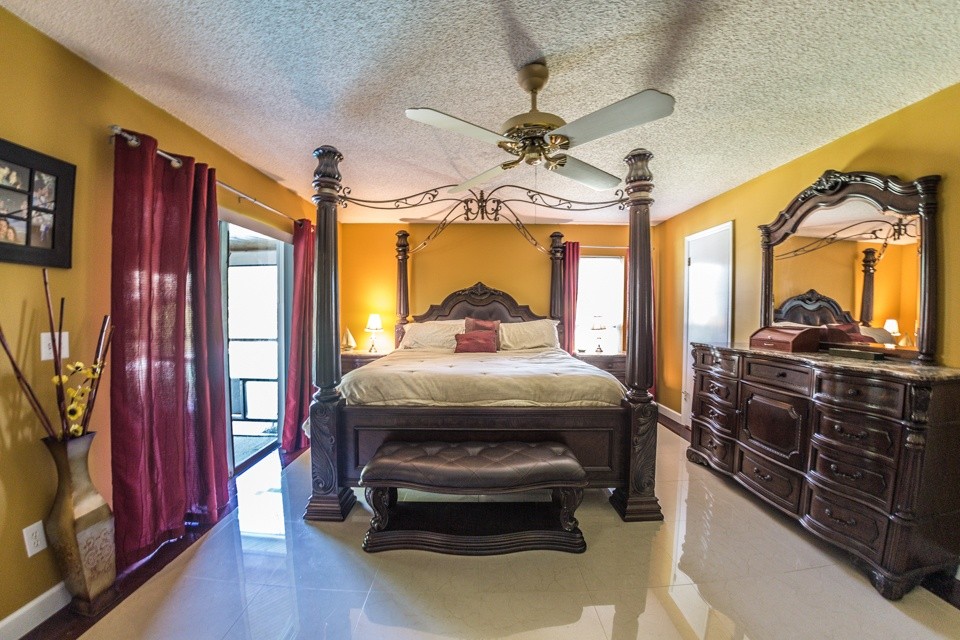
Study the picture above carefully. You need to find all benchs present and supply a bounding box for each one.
[357,438,588,555]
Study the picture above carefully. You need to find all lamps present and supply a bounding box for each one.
[363,312,383,353]
[884,318,900,337]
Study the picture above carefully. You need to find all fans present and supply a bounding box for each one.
[404,63,676,193]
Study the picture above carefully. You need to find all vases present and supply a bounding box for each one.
[40,431,123,616]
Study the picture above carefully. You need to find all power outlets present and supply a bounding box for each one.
[21,520,48,559]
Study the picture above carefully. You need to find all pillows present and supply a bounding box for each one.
[399,318,562,354]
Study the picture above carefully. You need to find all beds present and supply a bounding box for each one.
[772,218,922,327]
[300,144,666,523]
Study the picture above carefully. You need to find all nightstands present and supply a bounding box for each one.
[574,349,627,385]
[339,350,391,376]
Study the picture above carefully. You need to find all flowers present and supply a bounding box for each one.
[0,266,115,439]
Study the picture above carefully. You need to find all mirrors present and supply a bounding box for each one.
[757,170,941,364]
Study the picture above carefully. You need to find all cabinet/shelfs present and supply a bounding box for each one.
[686,341,960,601]
